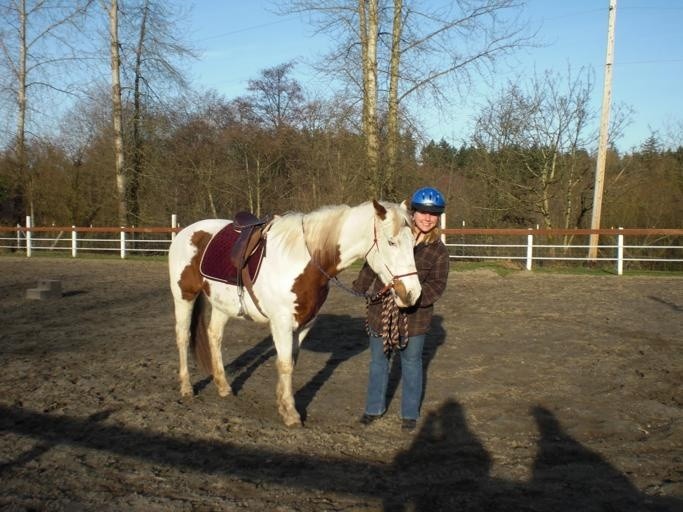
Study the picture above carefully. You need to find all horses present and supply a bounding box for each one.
[167,196,424,430]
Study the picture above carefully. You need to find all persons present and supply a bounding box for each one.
[351,185,449,432]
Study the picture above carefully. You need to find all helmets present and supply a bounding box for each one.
[412,187,445,215]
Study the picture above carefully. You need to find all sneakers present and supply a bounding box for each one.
[401,417,416,433]
[359,413,382,427]
